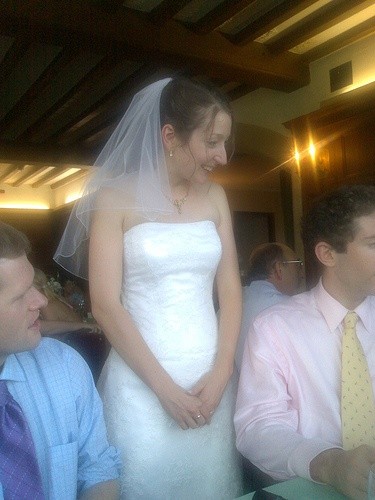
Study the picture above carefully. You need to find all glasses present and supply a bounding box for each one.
[281,257,303,272]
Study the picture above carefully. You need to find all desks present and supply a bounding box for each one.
[232,477,347,500]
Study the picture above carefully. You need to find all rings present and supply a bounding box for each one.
[196,415,202,418]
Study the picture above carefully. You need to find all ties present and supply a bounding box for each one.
[341,312,375,450]
[0,379,46,500]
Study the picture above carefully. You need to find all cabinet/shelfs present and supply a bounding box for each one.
[282,87,375,292]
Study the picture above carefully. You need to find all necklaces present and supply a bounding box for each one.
[154,181,192,209]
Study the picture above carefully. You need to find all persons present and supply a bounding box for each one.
[233,182,375,500]
[0,221,124,500]
[238,242,303,369]
[35,264,100,334]
[50,77,243,499]
